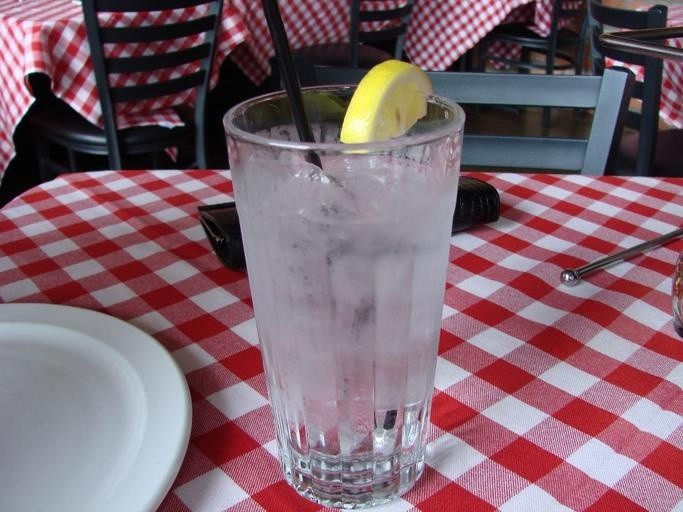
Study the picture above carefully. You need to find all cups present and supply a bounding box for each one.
[222,81,469,511]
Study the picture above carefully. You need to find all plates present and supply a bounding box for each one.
[0,302,194,512]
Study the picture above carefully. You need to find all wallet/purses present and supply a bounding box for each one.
[196,172,501,273]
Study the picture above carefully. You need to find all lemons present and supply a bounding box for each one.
[339,58,432,144]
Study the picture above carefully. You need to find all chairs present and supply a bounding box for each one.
[24,1,670,184]
